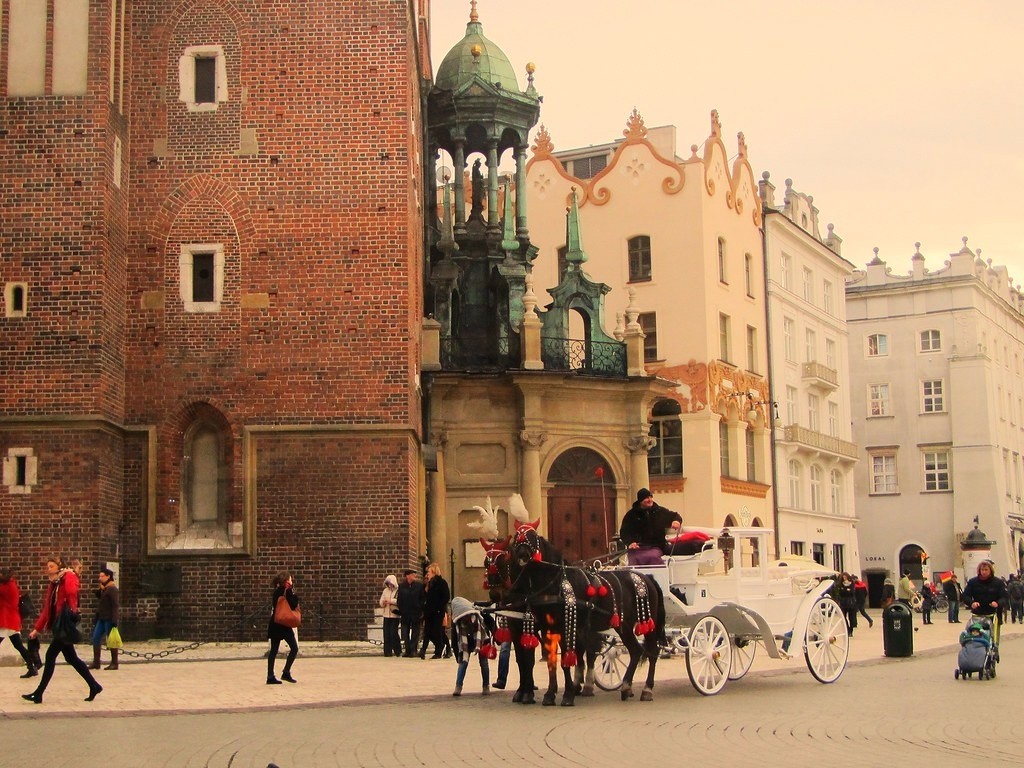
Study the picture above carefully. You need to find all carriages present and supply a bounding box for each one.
[465,493,849,706]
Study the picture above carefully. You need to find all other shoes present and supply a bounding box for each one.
[444,651,453,659]
[949,620,962,623]
[453,683,462,696]
[928,622,933,624]
[22,693,42,702]
[281,671,297,683]
[492,681,506,689]
[430,653,444,660]
[85,683,103,701]
[869,620,873,627]
[266,676,282,683]
[402,653,413,657]
[483,684,492,695]
[533,685,538,690]
[21,667,39,678]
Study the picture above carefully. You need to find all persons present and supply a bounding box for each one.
[839,572,873,637]
[898,569,916,607]
[417,563,453,659]
[619,487,682,565]
[880,578,895,604]
[1002,570,1024,623]
[22,554,103,703]
[86,568,119,670]
[0,567,38,678]
[450,596,500,696]
[921,581,933,624]
[492,640,538,690]
[944,575,963,623]
[266,571,299,684]
[397,570,425,656]
[959,624,990,642]
[779,631,793,656]
[379,575,402,656]
[962,560,1009,646]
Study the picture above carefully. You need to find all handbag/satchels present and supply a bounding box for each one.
[274,587,302,627]
[19,592,34,616]
[106,626,123,648]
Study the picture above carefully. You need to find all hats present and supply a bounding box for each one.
[638,487,653,502]
[923,581,932,586]
[405,569,416,574]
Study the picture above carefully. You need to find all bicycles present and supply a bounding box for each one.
[912,591,951,613]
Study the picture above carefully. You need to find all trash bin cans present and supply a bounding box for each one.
[882,602,913,659]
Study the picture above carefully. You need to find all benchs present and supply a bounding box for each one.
[626,539,713,583]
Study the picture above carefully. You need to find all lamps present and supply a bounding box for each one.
[753,400,782,427]
[728,391,757,418]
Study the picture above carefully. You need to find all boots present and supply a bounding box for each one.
[88,643,102,670]
[104,647,120,670]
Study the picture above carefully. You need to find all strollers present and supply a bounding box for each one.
[955,603,999,681]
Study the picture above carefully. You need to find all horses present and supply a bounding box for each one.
[479,535,606,704]
[512,517,668,707]
